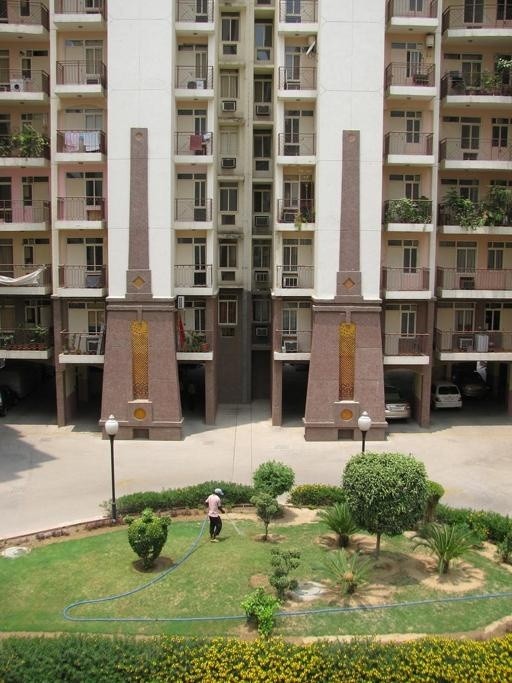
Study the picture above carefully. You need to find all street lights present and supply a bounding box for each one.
[104,411,122,524]
[358,411,370,455]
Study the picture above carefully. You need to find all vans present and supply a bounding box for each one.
[432,379,464,412]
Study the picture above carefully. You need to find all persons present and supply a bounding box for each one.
[204,488,227,542]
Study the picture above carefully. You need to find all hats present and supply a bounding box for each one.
[214,487,225,497]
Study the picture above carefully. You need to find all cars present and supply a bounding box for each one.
[384,381,413,422]
[0,385,20,416]
[457,371,491,399]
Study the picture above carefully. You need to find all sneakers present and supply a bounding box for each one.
[210,538,219,543]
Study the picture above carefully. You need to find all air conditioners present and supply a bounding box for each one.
[286,82,301,90]
[221,100,237,113]
[284,211,298,223]
[186,80,203,89]
[458,337,474,350]
[254,216,269,227]
[284,340,298,353]
[177,295,185,309]
[254,104,271,115]
[256,271,268,282]
[84,78,101,85]
[255,326,268,337]
[220,158,237,168]
[10,79,25,92]
[283,276,299,287]
[425,35,435,48]
[306,35,316,47]
[87,340,98,352]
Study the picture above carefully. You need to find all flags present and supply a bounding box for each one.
[178,314,185,349]
